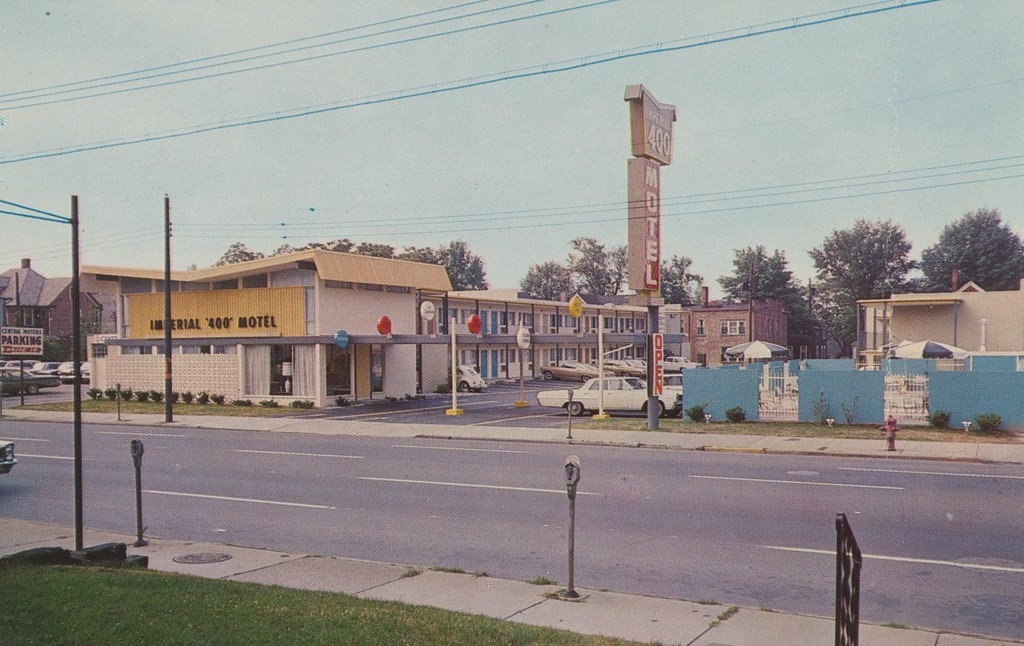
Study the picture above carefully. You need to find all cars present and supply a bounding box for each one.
[536,377,684,419]
[0,441,18,474]
[0,360,92,384]
[589,356,704,400]
[0,367,62,395]
[539,362,615,382]
[449,365,489,393]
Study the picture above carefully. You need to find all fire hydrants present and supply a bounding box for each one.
[880,414,902,452]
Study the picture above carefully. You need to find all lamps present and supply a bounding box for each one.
[283,362,293,393]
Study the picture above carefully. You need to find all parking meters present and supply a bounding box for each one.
[128,436,152,549]
[562,455,583,598]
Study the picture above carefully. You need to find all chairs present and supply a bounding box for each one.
[885,397,924,416]
[759,389,798,412]
